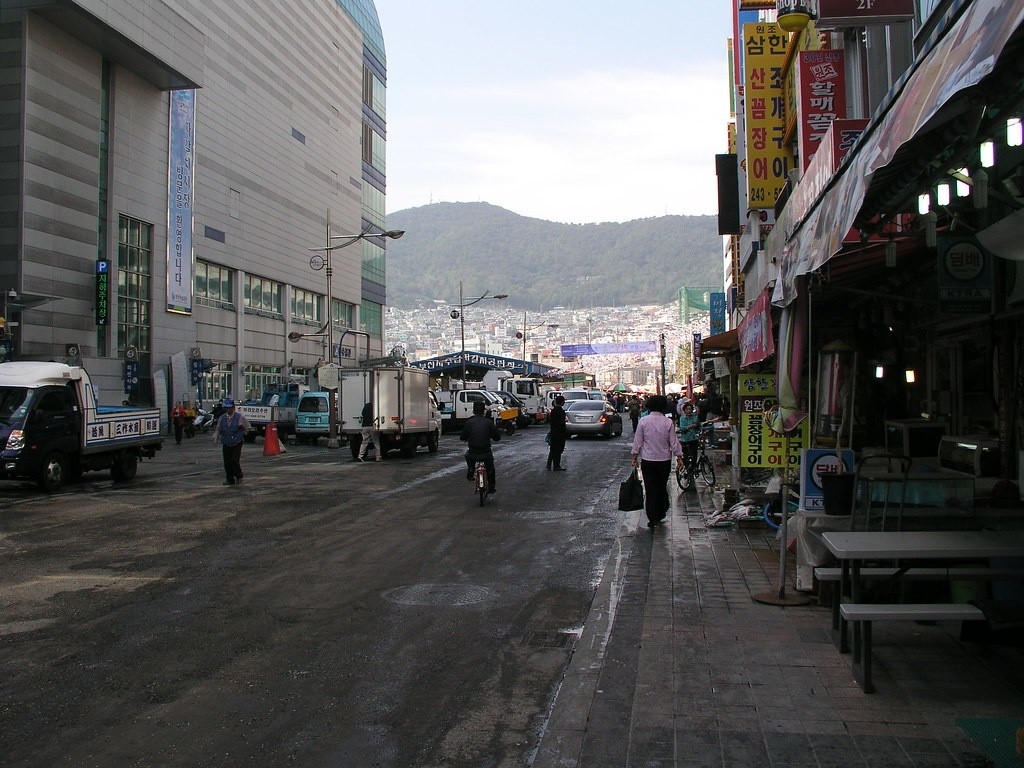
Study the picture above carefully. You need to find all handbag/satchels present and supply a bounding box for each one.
[618,468,644,512]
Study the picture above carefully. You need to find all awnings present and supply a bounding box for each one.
[696,326,739,359]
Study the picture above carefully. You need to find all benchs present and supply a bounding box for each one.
[815,568,985,698]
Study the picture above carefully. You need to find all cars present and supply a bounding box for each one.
[564,400,622,440]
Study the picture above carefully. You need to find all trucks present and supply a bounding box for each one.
[1,361,164,496]
[337,365,445,460]
[234,382,311,446]
[435,371,609,437]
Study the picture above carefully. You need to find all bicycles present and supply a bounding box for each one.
[675,426,716,491]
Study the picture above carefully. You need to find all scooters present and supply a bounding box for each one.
[192,408,214,433]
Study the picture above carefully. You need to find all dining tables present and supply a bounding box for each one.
[823,533,1024,662]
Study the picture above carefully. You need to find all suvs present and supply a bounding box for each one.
[295,391,348,446]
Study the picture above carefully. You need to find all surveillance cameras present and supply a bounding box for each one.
[9,291,17,299]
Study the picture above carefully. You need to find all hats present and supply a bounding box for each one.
[473,401,488,410]
[223,399,233,408]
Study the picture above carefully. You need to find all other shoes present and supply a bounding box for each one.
[359,457,364,462]
[376,459,383,462]
[546,466,566,471]
[224,477,242,486]
[646,520,661,527]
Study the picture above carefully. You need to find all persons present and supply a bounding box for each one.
[357,401,384,462]
[607,390,650,434]
[183,401,197,437]
[213,399,225,420]
[170,401,187,446]
[545,395,567,471]
[459,401,502,495]
[665,382,730,485]
[191,400,200,416]
[630,396,685,527]
[213,397,252,485]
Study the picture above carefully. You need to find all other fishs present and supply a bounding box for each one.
[702,498,764,528]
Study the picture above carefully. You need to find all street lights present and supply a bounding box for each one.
[516,312,561,379]
[449,280,509,389]
[288,207,405,449]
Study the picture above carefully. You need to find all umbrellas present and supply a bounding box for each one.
[608,382,646,394]
[763,284,809,598]
[686,373,694,400]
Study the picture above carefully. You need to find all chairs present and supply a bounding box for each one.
[853,455,912,532]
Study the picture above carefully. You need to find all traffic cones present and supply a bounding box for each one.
[261,423,276,455]
[271,423,281,454]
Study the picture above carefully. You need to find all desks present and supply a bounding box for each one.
[858,470,975,532]
[884,420,950,456]
